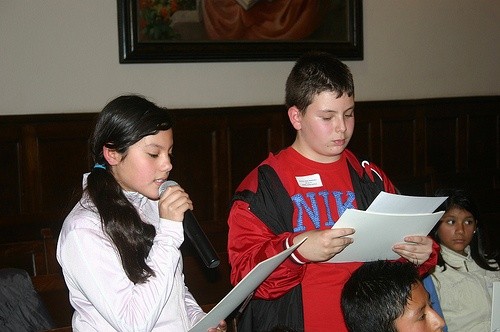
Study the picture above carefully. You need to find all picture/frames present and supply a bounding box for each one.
[117,0,363,64]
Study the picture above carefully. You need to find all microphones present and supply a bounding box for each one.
[159,180,220,268]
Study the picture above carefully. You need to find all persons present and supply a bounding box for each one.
[56,95,228,332]
[423,190,500,332]
[341,257,446,332]
[227,49,440,332]
